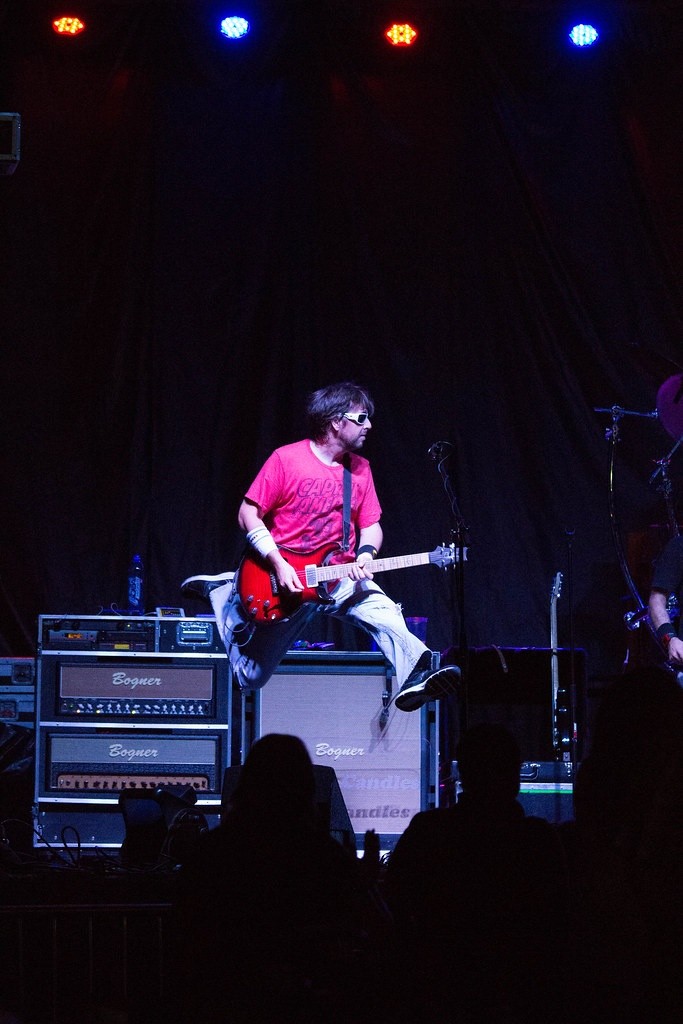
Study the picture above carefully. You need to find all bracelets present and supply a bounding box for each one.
[247,527,280,558]
[356,544,378,558]
[656,624,677,645]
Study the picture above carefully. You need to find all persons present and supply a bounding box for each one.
[199,535,682,934]
[180,382,467,712]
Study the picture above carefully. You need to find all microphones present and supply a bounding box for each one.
[428,442,443,461]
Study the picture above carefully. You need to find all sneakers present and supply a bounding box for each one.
[394,666,462,713]
[181,571,238,597]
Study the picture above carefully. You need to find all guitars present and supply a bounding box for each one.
[234,541,468,628]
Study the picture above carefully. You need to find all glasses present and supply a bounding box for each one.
[338,411,370,425]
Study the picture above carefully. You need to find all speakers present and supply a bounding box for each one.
[442,646,589,784]
[252,665,428,851]
[221,766,356,851]
[120,783,216,873]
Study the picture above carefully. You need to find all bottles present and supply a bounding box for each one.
[128,553,145,616]
